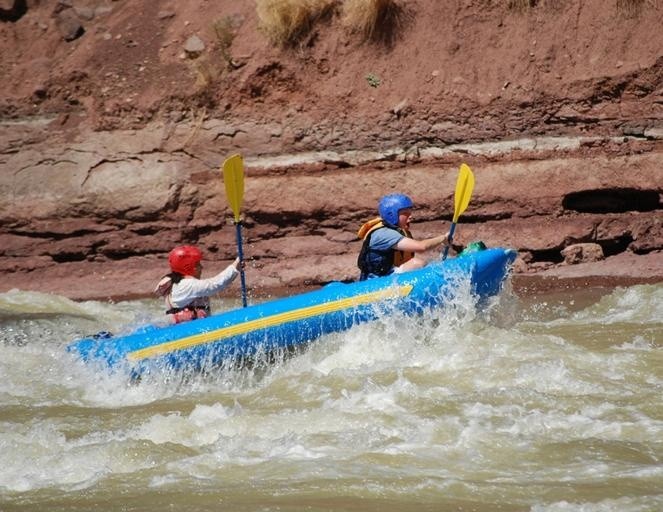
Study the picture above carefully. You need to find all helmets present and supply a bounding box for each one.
[169,244,203,276]
[378,192,413,226]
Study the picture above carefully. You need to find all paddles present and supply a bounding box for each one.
[222,155,248,307]
[441,163,474,260]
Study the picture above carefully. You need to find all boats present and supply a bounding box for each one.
[66,248,517,379]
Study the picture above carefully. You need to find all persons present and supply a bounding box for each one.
[152,244,247,325]
[354,193,459,283]
